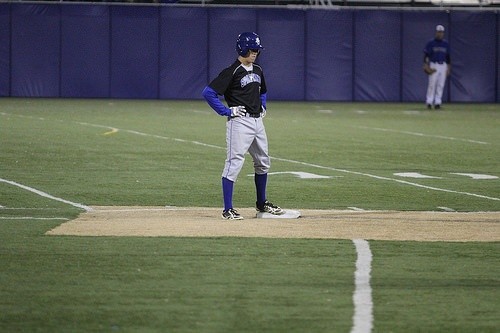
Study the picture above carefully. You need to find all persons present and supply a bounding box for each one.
[418,25,452,110]
[202,31,283,220]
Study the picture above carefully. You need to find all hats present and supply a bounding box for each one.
[435,24,445,32]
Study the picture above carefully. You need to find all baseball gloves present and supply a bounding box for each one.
[424,65,437,75]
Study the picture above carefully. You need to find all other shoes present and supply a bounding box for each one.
[435,104,440,109]
[255,201,285,216]
[426,104,432,110]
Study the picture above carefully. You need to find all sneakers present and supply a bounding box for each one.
[222,209,244,221]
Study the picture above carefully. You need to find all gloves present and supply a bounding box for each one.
[260,106,269,120]
[447,63,451,76]
[422,62,433,75]
[229,105,247,118]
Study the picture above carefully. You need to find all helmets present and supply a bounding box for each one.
[235,33,264,55]
